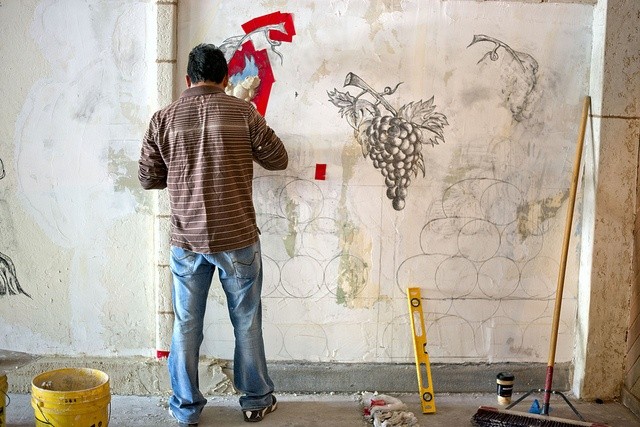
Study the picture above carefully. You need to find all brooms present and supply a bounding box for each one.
[468,94,624,427]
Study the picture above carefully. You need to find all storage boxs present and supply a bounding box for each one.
[0,369,10,427]
[30,366,113,427]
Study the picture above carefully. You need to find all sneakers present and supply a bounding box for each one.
[243,395,276,421]
[168,406,198,427]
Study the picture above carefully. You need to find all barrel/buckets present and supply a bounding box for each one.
[0,371,10,427]
[29,368,112,427]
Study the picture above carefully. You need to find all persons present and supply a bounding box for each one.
[136,42,291,427]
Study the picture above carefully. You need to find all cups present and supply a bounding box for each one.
[496,372,515,406]
